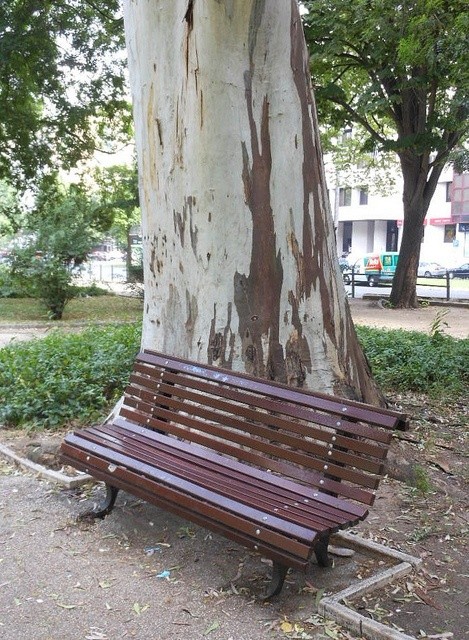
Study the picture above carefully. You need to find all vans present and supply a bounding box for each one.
[343,252,400,287]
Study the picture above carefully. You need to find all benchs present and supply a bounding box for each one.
[60,347,410,603]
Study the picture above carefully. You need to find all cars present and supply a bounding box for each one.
[447,263,469,279]
[417,262,446,278]
[1,235,49,263]
